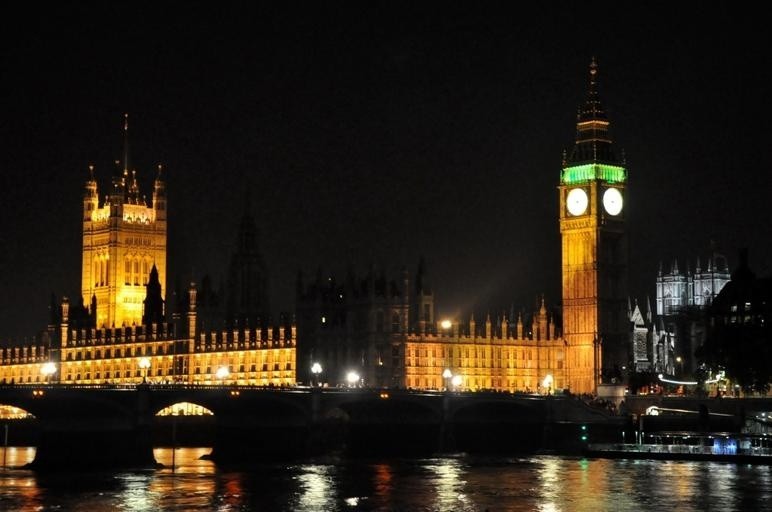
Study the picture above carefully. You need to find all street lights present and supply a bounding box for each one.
[39,362,57,384]
[139,357,152,385]
[215,366,230,385]
[310,362,324,386]
[441,368,453,391]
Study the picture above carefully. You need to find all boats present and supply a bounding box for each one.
[578,429,772,462]
